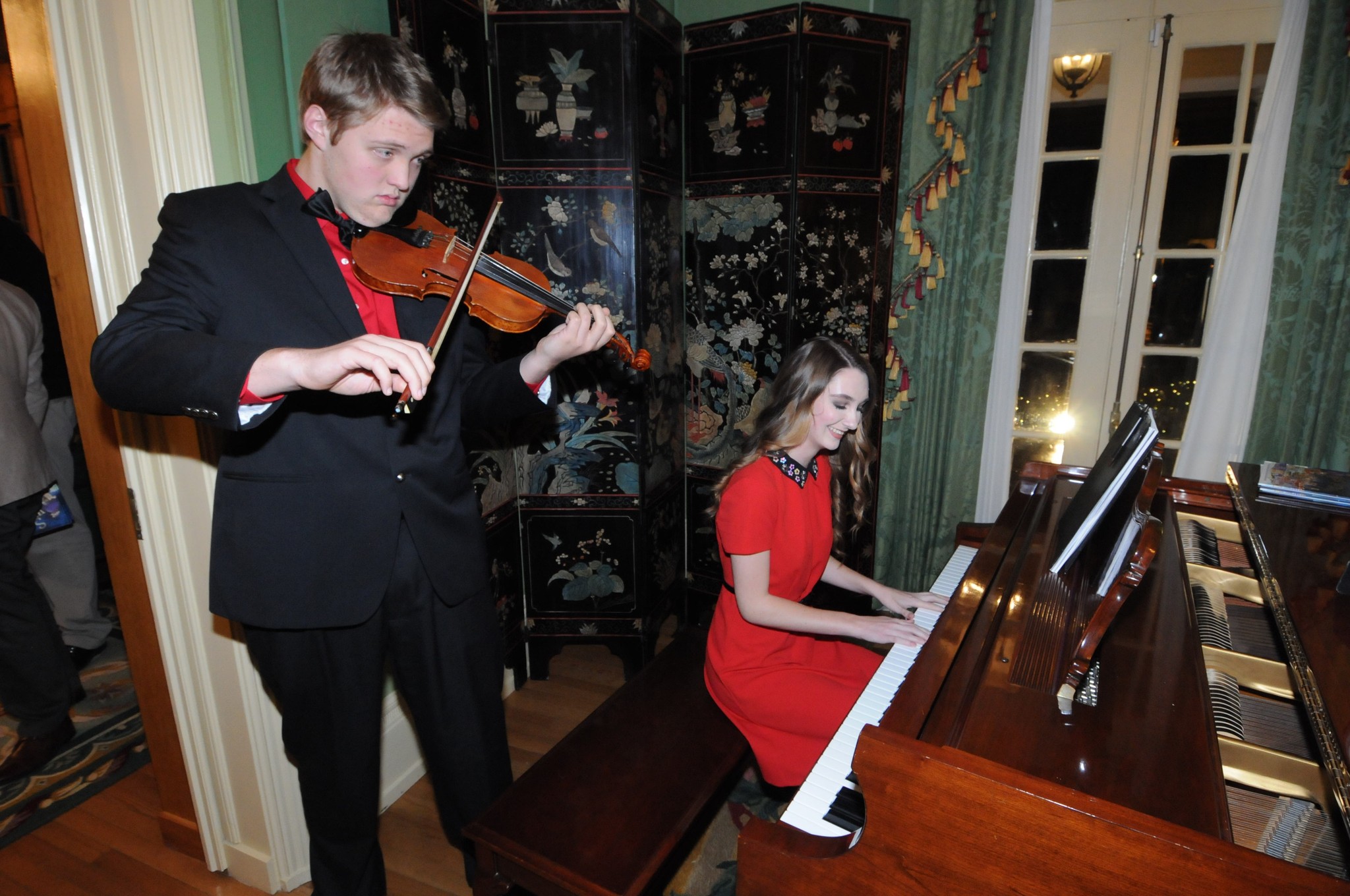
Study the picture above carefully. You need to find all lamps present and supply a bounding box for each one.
[1053,53,1104,99]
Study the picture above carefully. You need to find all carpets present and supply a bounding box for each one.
[0,591,162,848]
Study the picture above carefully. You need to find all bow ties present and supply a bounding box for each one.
[301,187,355,251]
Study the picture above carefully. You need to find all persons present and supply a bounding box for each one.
[88,30,615,896]
[0,280,90,789]
[703,335,953,788]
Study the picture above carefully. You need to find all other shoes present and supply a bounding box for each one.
[4,685,84,719]
[67,642,107,672]
[0,718,77,779]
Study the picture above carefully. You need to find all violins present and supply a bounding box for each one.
[350,203,652,371]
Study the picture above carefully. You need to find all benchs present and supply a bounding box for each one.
[462,594,772,895]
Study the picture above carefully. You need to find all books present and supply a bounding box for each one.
[1257,460,1350,507]
[1047,400,1160,574]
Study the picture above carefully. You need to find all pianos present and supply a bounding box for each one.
[737,443,1350,896]
